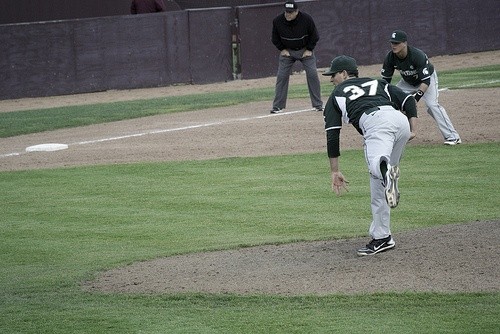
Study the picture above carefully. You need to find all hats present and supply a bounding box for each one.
[322,56,358,75]
[390,30,408,43]
[283,1,298,11]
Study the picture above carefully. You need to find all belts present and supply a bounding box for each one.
[365,106,399,115]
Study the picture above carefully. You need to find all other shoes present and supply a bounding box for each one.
[316,105,323,111]
[270,107,285,114]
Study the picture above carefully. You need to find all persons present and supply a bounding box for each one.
[381,30,461,146]
[270,0,324,114]
[322,55,418,255]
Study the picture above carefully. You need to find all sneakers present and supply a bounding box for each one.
[357,235,396,256]
[444,136,461,145]
[383,166,401,209]
